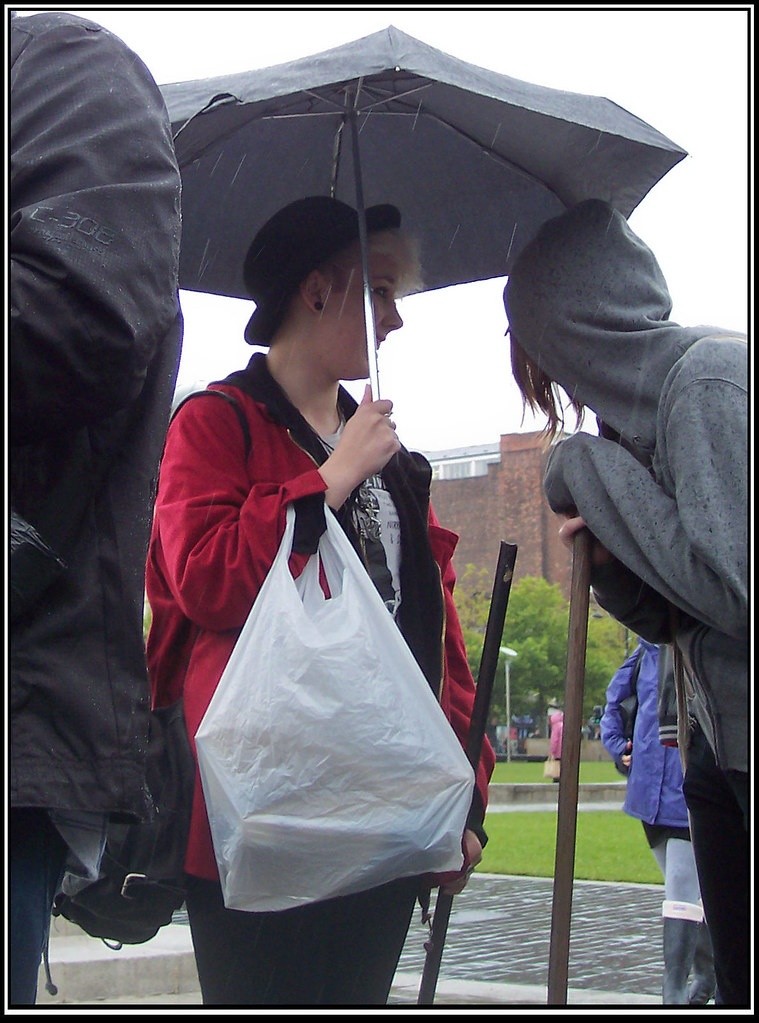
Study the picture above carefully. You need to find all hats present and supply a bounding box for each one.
[243,195,402,346]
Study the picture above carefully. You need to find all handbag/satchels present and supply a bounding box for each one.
[51,694,193,950]
[544,754,561,780]
[193,501,477,913]
[614,646,647,777]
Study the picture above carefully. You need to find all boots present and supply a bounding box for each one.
[688,922,716,1004]
[660,900,704,1004]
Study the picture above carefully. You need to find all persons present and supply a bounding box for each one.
[10,10,184,1006]
[504,198,746,1008]
[601,639,717,1005]
[149,194,497,1009]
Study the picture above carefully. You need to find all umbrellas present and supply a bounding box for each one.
[158,26,689,479]
